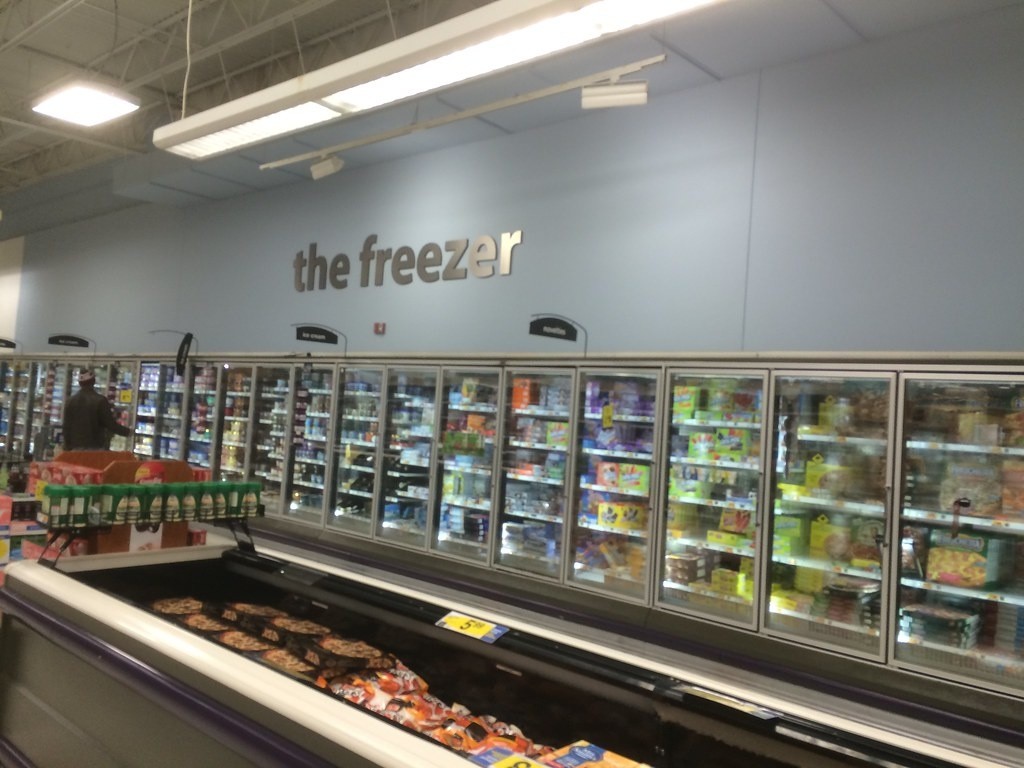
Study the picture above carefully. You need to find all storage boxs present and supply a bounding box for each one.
[808,513,858,549]
[770,565,881,630]
[808,548,832,561]
[776,452,1024,524]
[536,739,640,768]
[384,377,656,583]
[666,528,705,539]
[774,516,809,539]
[707,529,744,547]
[898,526,1024,654]
[0,449,211,587]
[718,509,750,534]
[668,503,698,530]
[773,533,805,556]
[849,558,881,570]
[669,378,763,505]
[798,386,1024,449]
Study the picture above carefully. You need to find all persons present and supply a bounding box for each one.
[62,368,135,451]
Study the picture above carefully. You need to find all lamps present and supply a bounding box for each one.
[310,154,344,180]
[581,76,649,109]
[30,80,142,127]
[154,0,725,162]
